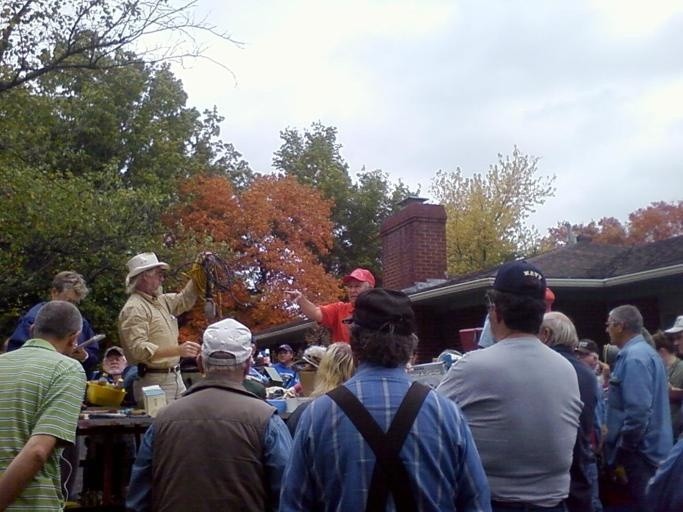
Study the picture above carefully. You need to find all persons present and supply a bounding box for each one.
[0,301,88,512]
[124,318,294,512]
[280,289,493,511]
[0,249,419,419]
[432,260,683,512]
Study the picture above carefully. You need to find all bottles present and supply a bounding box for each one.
[97,371,107,385]
[116,377,123,388]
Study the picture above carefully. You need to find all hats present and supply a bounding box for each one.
[343,268,375,288]
[278,344,292,351]
[126,251,168,286]
[354,287,417,336]
[105,347,124,357]
[664,316,683,333]
[574,339,599,354]
[490,260,546,299]
[203,318,252,366]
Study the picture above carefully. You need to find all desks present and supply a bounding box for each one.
[72,408,159,510]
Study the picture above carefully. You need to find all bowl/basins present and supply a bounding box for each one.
[265,399,286,413]
[86,381,125,407]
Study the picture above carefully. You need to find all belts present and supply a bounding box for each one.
[148,364,180,373]
